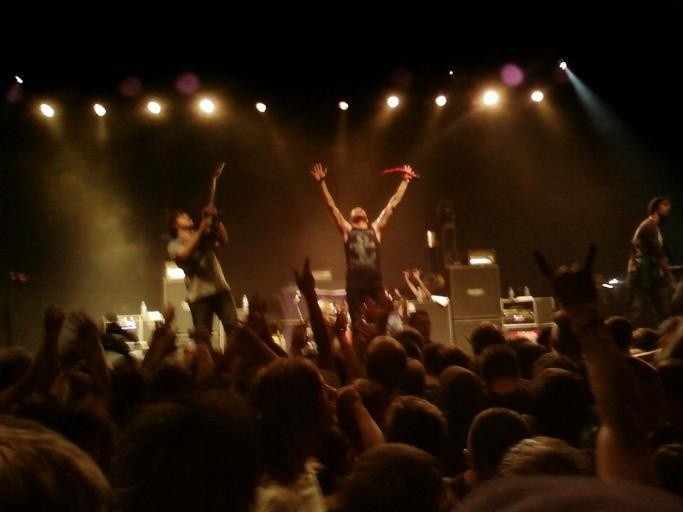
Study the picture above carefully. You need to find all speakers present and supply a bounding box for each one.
[405,298,454,349]
[162,279,219,333]
[454,320,503,361]
[449,264,501,320]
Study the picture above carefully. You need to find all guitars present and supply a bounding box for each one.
[194,164,233,249]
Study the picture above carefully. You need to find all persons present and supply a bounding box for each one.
[308,161,416,335]
[165,204,240,357]
[0,244,683,512]
[628,196,678,325]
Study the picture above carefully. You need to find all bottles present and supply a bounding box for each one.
[508,286,516,299]
[239,293,250,310]
[523,285,531,297]
[140,301,149,317]
